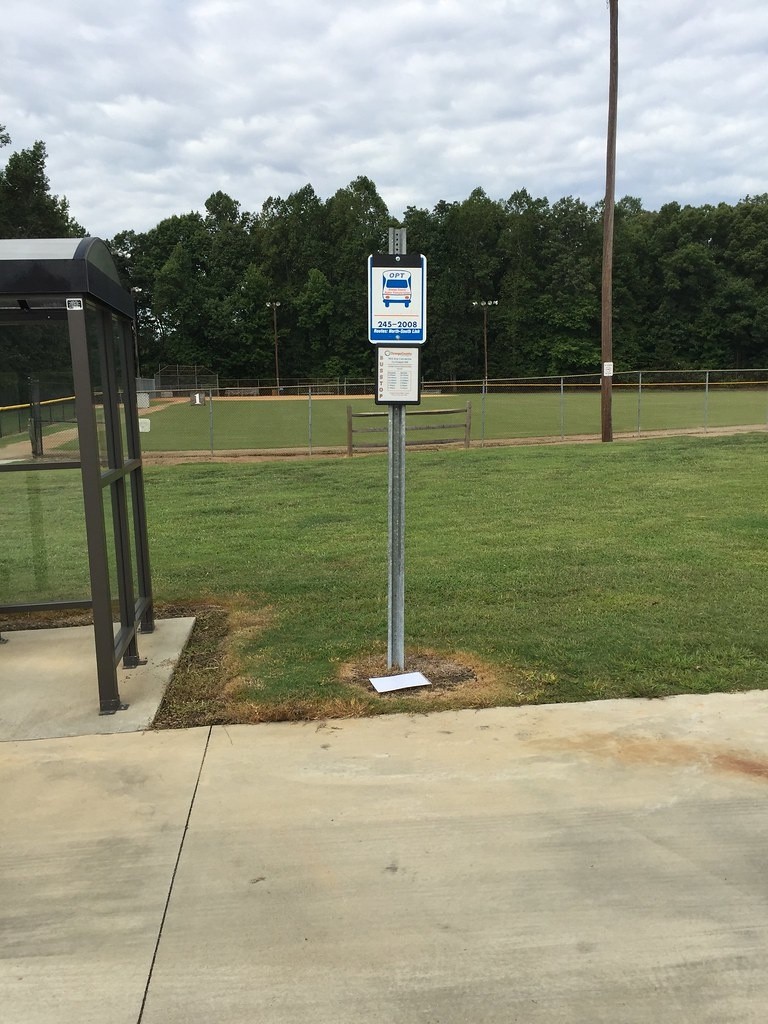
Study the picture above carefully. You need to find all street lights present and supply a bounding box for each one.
[471,300,500,396]
[266,301,284,398]
